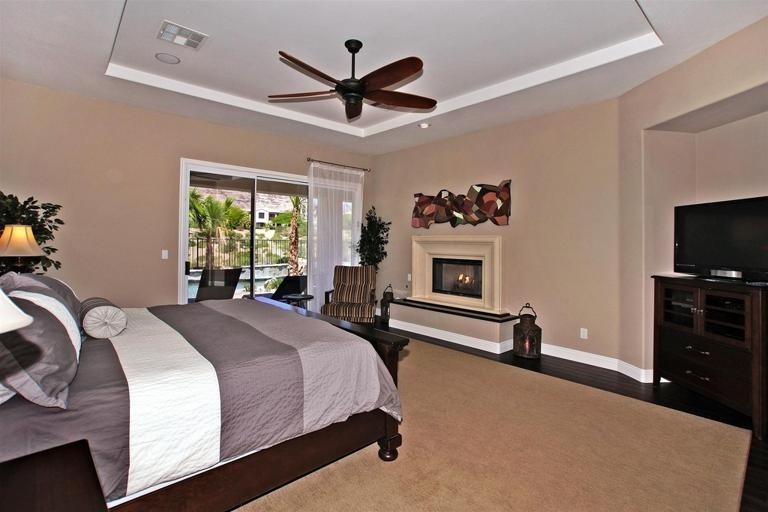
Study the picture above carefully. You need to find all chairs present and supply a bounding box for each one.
[320,265,390,331]
[188,268,242,304]
[242,276,307,304]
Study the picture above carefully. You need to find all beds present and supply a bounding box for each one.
[0,307,410,512]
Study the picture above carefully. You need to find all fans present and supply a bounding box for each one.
[267,39,438,120]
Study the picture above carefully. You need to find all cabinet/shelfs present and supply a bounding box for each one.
[649,274,768,441]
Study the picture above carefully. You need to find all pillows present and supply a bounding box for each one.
[0,272,127,409]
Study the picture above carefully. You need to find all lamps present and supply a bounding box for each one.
[513,303,542,359]
[0,224,45,274]
[380,285,395,322]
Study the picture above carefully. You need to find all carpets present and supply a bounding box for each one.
[231,337,754,512]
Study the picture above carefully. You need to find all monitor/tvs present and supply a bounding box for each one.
[673,195,768,284]
